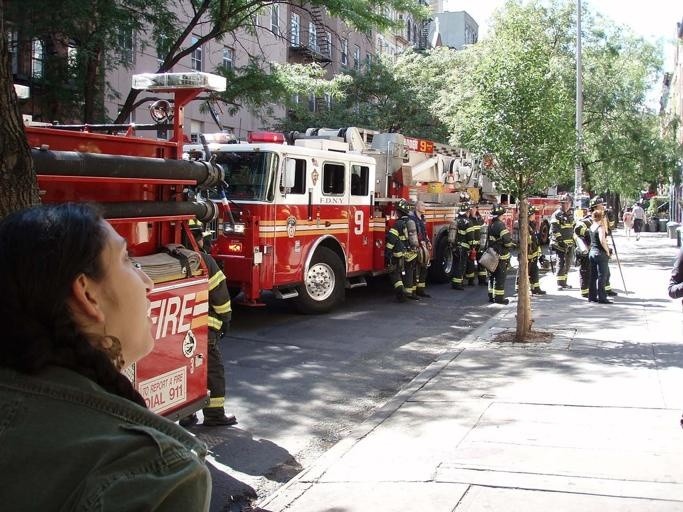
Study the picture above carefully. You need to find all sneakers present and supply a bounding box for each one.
[203,414,237,428]
[556,284,572,292]
[582,290,617,305]
[397,291,432,306]
[487,298,509,306]
[451,280,488,291]
[532,289,546,295]
[179,412,198,428]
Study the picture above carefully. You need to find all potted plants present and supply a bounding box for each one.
[646,196,658,231]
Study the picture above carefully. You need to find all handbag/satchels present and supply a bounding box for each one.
[478,246,500,274]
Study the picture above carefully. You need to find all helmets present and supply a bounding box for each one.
[557,193,574,204]
[490,205,507,215]
[394,197,412,212]
[460,201,470,211]
[527,203,535,216]
[588,196,607,207]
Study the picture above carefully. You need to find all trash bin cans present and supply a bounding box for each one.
[659,218,669,232]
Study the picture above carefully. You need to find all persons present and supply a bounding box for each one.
[385,194,647,304]
[669,248,682,299]
[0,203,213,512]
[180,219,236,428]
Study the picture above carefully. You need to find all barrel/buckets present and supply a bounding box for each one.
[467,187,480,201]
[648,216,682,247]
[427,181,442,193]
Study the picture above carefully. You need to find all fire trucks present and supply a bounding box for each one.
[181,127,514,317]
[22,70,227,421]
[495,176,598,247]
